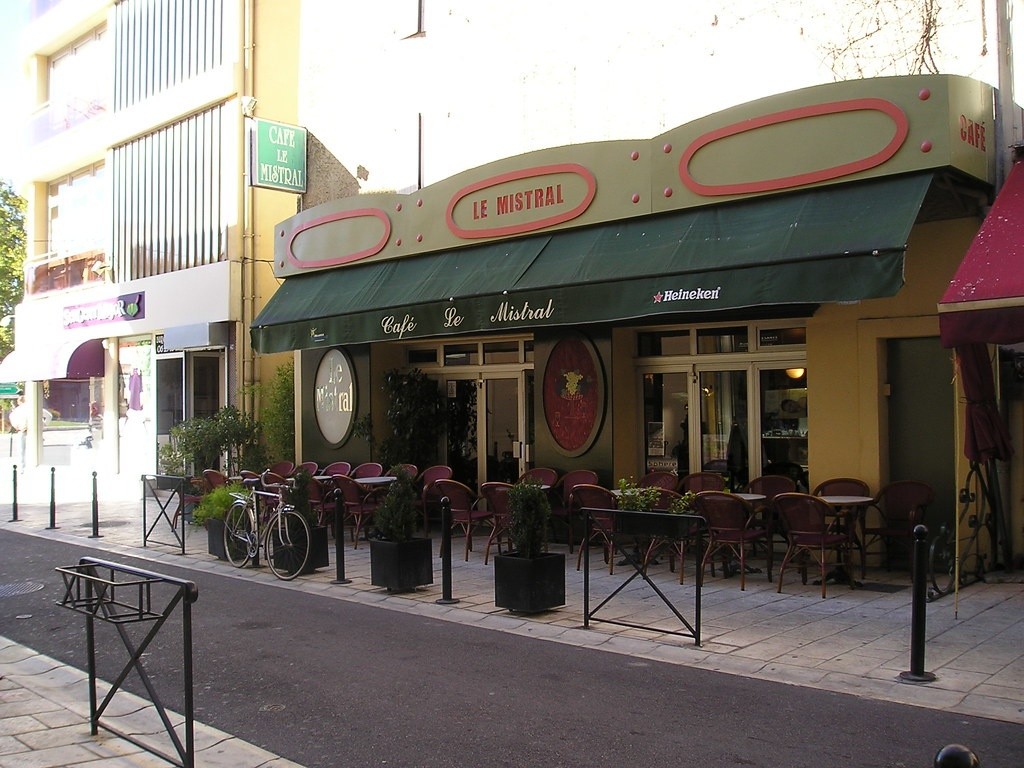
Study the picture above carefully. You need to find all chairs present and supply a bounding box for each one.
[183,461,935,599]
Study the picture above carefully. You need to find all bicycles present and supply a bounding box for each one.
[222,470,312,582]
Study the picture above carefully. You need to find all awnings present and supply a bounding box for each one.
[0,337,105,383]
[937,158,1024,349]
[248,171,936,356]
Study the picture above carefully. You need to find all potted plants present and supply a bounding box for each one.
[368,463,432,592]
[268,465,329,575]
[616,477,695,537]
[494,475,567,612]
[191,480,256,562]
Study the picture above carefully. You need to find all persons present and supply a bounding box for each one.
[9,395,52,474]
[781,397,806,417]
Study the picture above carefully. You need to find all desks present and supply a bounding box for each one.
[355,478,400,541]
[812,495,875,588]
[605,488,661,566]
[764,435,807,464]
[285,475,341,540]
[509,484,550,552]
[720,493,768,580]
